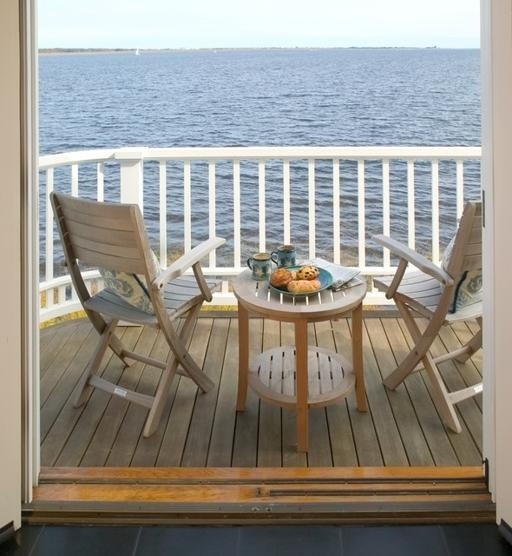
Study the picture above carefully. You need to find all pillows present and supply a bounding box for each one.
[437,225,485,321]
[94,242,171,314]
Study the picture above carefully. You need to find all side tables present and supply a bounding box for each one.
[231,242,369,452]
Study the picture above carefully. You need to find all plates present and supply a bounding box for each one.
[268,265,334,298]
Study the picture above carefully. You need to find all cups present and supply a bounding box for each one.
[270,245,296,268]
[246,252,272,281]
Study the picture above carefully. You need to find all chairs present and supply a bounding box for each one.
[50,188,224,435]
[373,203,490,434]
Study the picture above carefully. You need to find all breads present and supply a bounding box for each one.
[270,265,321,294]
